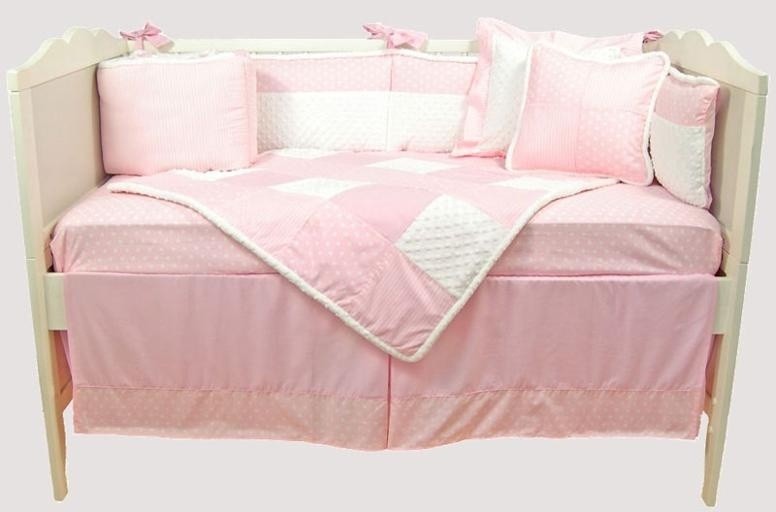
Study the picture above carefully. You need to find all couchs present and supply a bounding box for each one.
[6,24,768,507]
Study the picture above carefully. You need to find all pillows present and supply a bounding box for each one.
[461,24,662,159]
[505,42,671,186]
[391,50,477,157]
[258,56,391,154]
[100,53,258,176]
[649,65,720,210]
